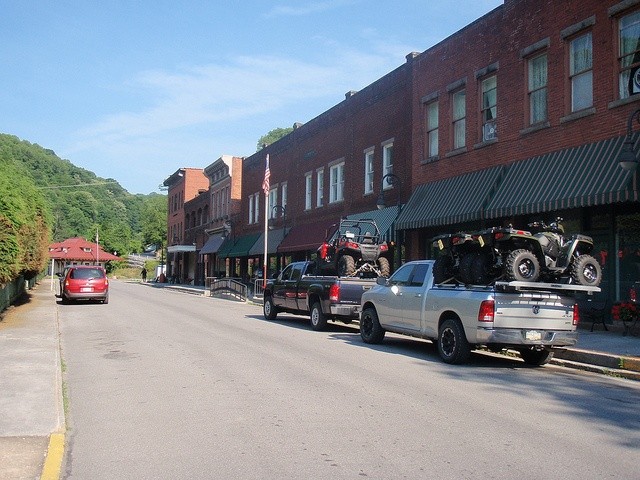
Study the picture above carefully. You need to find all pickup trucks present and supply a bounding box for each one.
[263,261,383,329]
[359,260,601,365]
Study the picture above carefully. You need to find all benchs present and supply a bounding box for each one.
[589,298,640,337]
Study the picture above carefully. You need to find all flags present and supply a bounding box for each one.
[261,157,271,197]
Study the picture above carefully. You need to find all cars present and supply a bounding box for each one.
[57,264,86,295]
[64,264,110,304]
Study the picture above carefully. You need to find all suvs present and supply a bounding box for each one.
[496,216,601,286]
[455,223,530,280]
[431,232,475,279]
[316,214,392,278]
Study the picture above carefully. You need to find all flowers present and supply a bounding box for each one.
[611,285,640,321]
